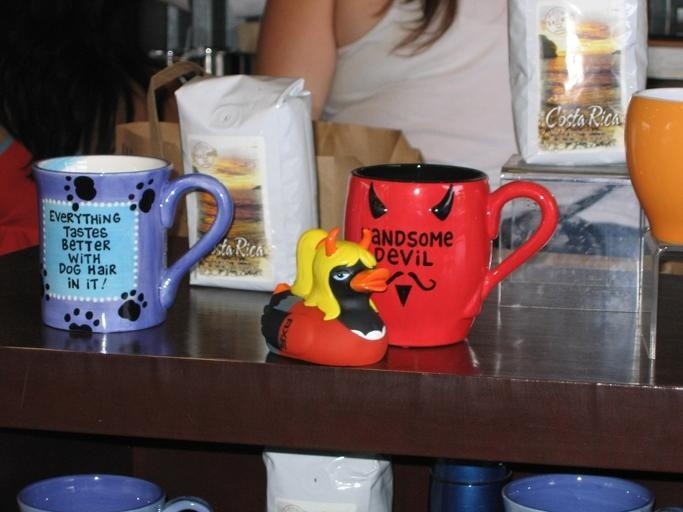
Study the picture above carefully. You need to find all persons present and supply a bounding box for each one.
[254,0,518,192]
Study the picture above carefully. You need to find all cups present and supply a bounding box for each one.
[426,459,511,512]
[17,473,212,512]
[500,472,683,512]
[624,85,683,246]
[32,154,233,332]
[343,161,560,350]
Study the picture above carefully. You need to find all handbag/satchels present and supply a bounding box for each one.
[112,59,421,296]
[507,1,649,173]
